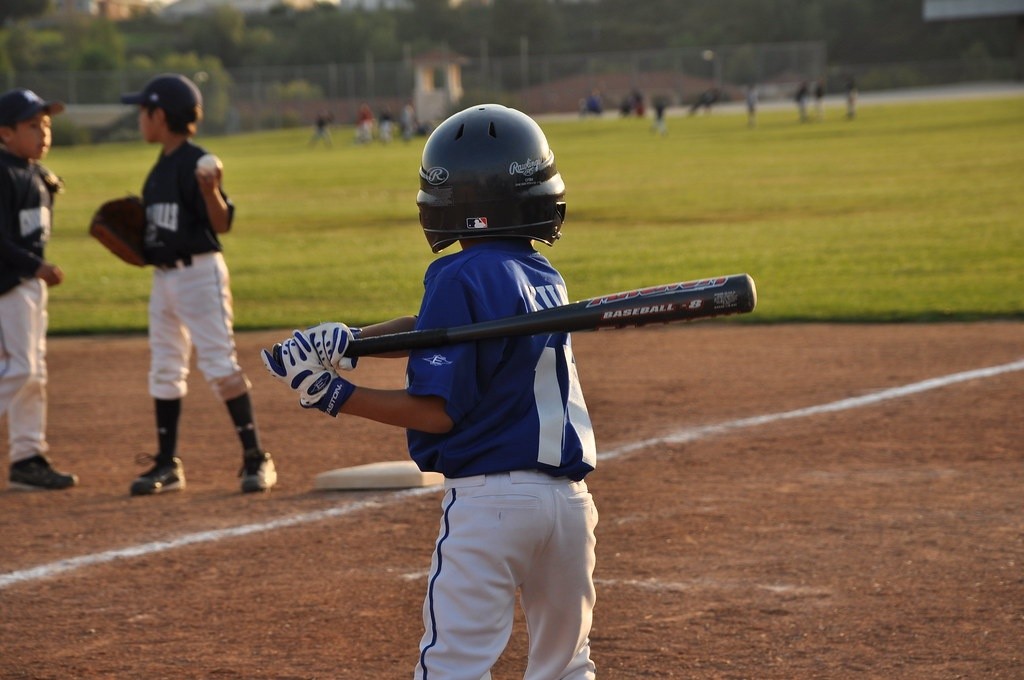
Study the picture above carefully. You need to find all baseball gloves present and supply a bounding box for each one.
[88,189,147,268]
[37,164,65,193]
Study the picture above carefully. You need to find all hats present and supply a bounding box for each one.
[119,74,203,113]
[0,88,64,125]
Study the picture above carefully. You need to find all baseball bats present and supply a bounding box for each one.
[271,272,760,366]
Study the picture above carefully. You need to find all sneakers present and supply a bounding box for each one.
[9,455,80,492]
[130,451,186,495]
[238,452,278,493]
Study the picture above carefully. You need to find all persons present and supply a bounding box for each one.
[795,77,828,123]
[688,83,729,116]
[842,71,858,120]
[0,88,79,490]
[307,105,337,149]
[578,88,671,136]
[260,104,599,680]
[745,81,761,126]
[354,103,437,145]
[89,73,277,496]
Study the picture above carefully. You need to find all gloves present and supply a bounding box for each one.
[260,329,356,418]
[303,322,362,371]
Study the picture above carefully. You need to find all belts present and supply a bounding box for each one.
[155,255,193,271]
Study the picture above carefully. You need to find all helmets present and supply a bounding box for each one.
[415,103,567,254]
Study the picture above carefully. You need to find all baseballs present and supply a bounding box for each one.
[196,153,223,177]
